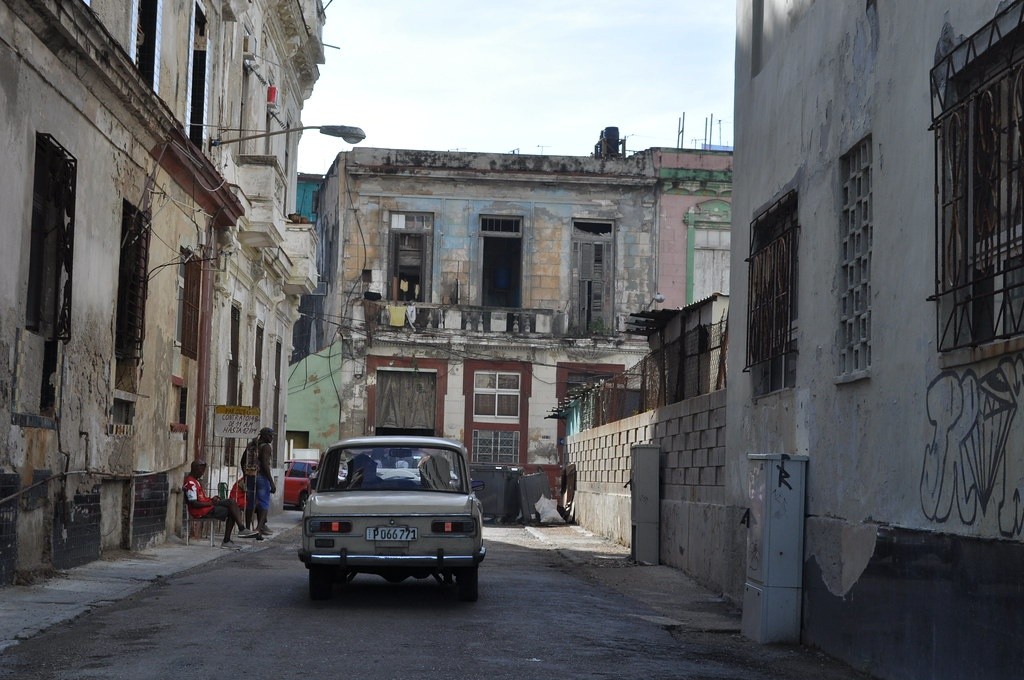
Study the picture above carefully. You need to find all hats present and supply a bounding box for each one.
[259,427,278,435]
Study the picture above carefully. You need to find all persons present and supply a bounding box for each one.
[182,458,261,551]
[229,472,265,525]
[239,426,277,536]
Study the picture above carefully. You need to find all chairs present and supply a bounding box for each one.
[183,494,221,550]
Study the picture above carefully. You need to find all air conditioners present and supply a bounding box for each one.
[267,86,281,107]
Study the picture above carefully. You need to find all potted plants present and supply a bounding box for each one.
[286,212,308,224]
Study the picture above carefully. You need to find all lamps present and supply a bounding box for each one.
[648,292,666,309]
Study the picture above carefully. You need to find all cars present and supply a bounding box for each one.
[298,436,486,603]
[229,460,345,511]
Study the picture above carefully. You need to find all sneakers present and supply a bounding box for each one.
[254,529,263,540]
[261,525,273,535]
[238,528,259,538]
[221,540,242,550]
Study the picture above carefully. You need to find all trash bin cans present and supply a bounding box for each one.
[470,462,523,523]
[517,472,551,524]
